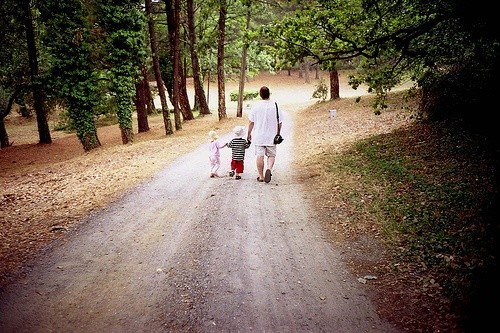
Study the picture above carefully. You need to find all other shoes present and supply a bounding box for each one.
[230,170,234,176]
[236,175,241,179]
[212,171,218,175]
[211,175,214,177]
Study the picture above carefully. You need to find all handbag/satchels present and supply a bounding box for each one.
[274,134,283,143]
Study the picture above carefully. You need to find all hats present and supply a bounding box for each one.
[208,130,219,141]
[233,126,246,138]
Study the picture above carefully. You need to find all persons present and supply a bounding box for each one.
[207,131,228,178]
[226,126,251,180]
[246,85,284,183]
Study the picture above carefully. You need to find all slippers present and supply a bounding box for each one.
[257,176,264,181]
[265,168,272,182]
